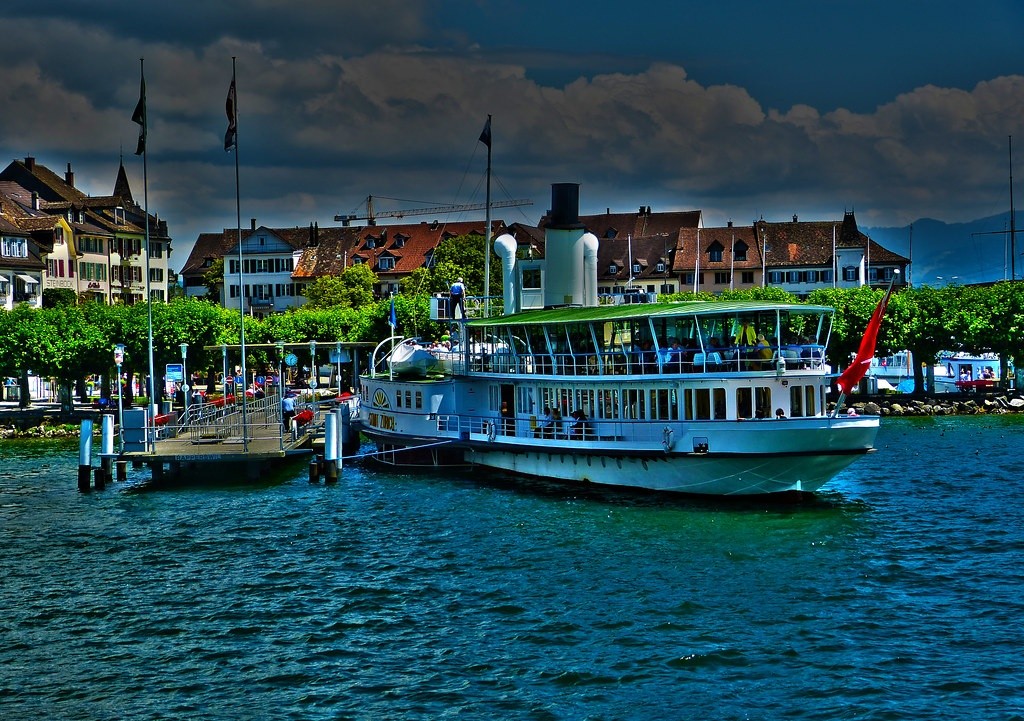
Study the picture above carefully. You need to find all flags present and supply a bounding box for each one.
[835,281,895,397]
[478,118,491,146]
[224,74,237,153]
[132,75,147,154]
[387,296,395,328]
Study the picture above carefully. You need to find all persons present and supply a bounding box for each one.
[847,408,861,417]
[534,407,590,441]
[960,366,995,382]
[92,393,117,409]
[247,384,264,399]
[282,389,296,433]
[640,326,824,372]
[776,408,788,419]
[450,277,466,320]
[407,326,491,370]
[192,389,208,409]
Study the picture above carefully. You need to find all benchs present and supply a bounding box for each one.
[530,431,623,440]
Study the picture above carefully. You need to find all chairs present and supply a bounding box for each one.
[655,348,828,373]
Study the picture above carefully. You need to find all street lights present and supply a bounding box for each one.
[219,341,228,423]
[335,342,342,398]
[113,345,124,452]
[308,340,317,426]
[178,343,190,432]
[273,340,285,450]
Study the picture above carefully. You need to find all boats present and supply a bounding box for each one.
[386,344,437,379]
[357,182,882,501]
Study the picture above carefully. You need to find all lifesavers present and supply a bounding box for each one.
[485,420,497,442]
[660,426,675,452]
